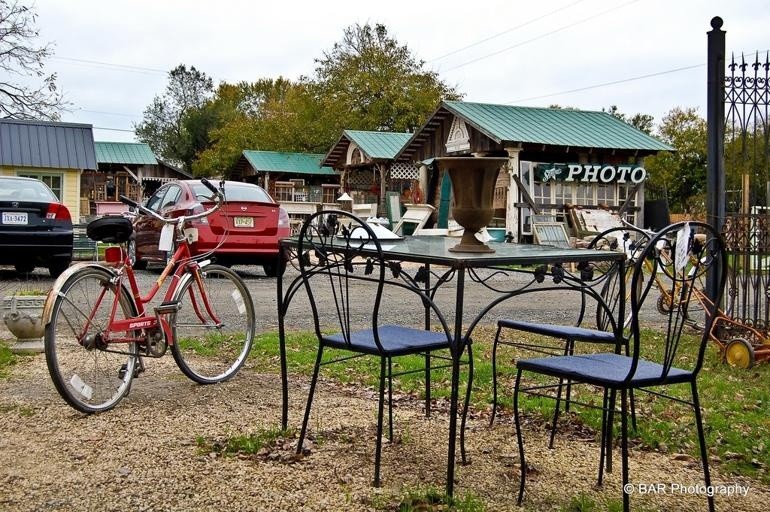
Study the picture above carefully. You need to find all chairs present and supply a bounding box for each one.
[292,210,730,512]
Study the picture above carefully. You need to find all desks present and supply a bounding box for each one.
[276,233,628,509]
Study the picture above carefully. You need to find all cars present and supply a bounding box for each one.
[0,176,74,279]
[126,178,291,276]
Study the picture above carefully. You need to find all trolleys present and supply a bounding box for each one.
[660,250,770,370]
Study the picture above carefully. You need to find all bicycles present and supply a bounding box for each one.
[40,177,255,413]
[597,205,736,333]
